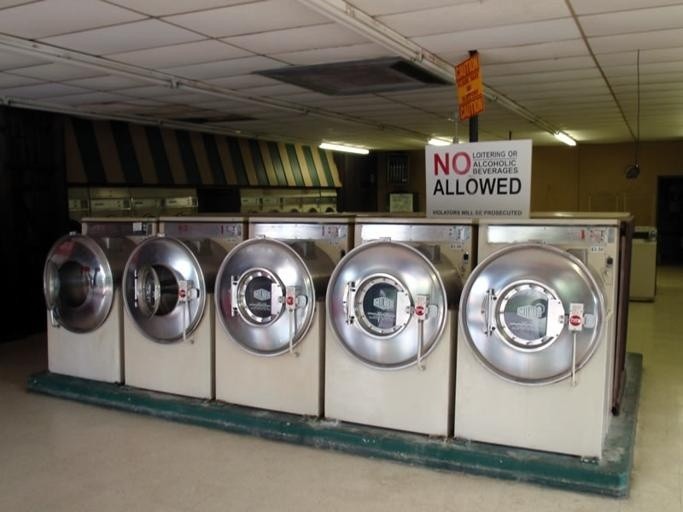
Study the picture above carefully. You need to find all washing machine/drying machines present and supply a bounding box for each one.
[454,209,634,461]
[322,213,474,442]
[42,187,245,404]
[212,211,351,421]
